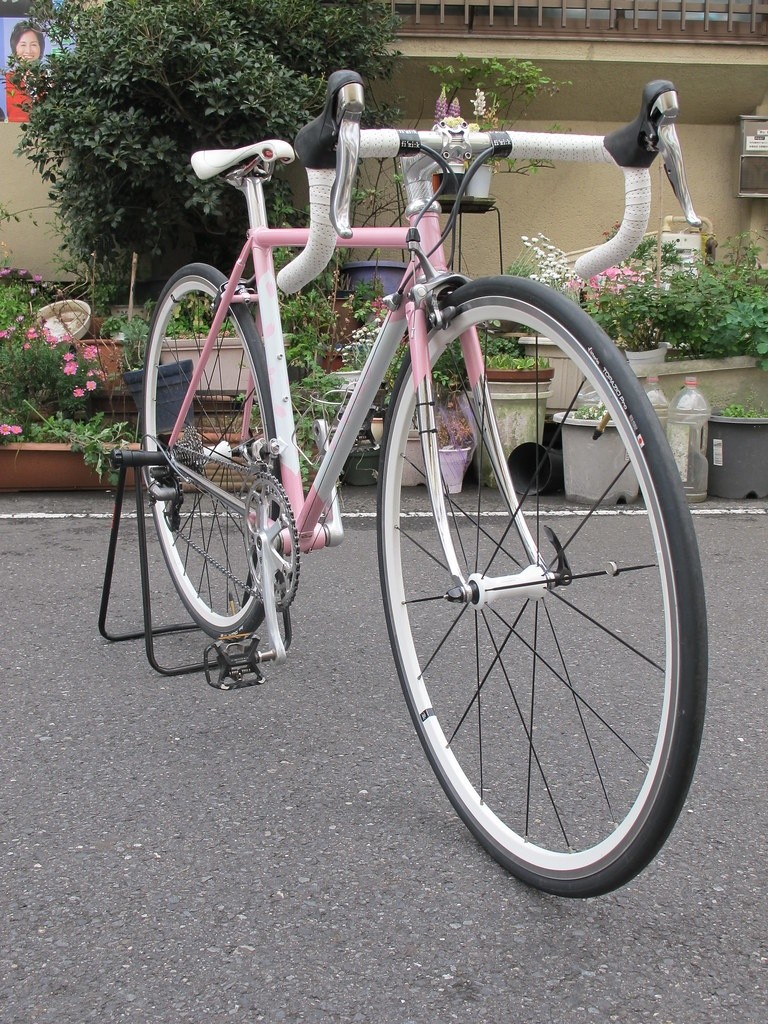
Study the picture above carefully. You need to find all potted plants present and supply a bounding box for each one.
[100,299,194,435]
[705,394,768,501]
[267,153,556,495]
[161,290,253,391]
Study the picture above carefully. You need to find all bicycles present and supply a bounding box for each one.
[95,66,709,899]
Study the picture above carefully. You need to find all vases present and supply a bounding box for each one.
[433,173,444,195]
[625,342,669,368]
[556,412,639,506]
[0,442,146,492]
[445,165,465,195]
[518,336,583,410]
[468,163,491,198]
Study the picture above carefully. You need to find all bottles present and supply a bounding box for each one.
[642,375,667,439]
[667,377,709,503]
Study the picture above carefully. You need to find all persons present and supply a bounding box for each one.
[6,21,46,122]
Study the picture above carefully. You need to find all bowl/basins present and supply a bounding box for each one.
[39,299,91,339]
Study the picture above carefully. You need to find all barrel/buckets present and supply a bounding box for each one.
[507,442,564,496]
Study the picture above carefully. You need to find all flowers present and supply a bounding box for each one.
[1,266,144,487]
[471,88,501,132]
[433,89,461,121]
[570,400,612,421]
[580,256,663,350]
